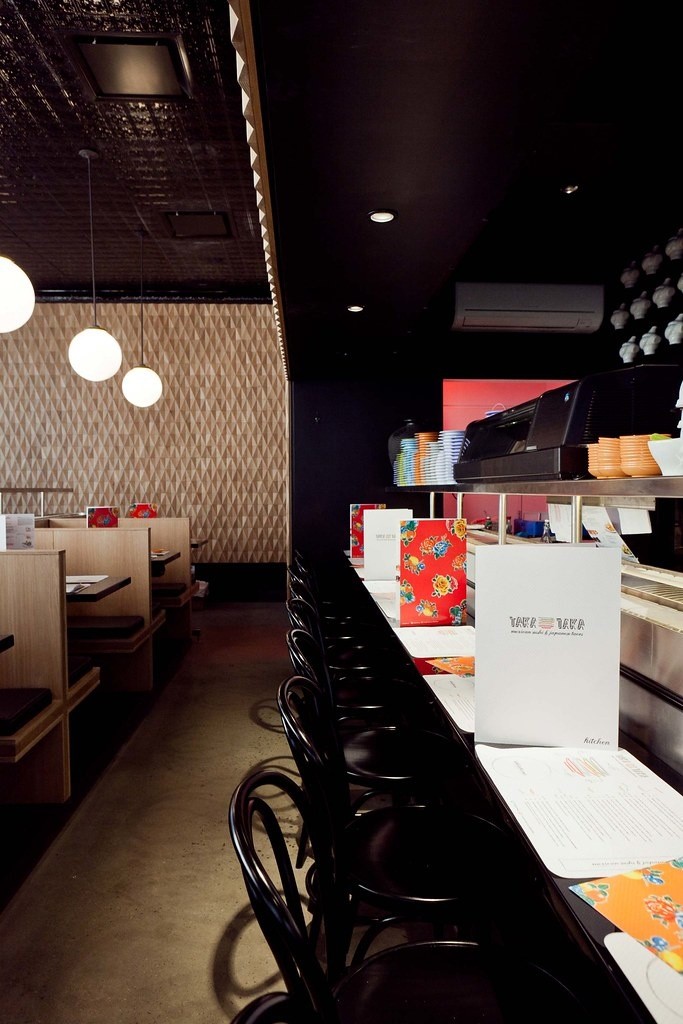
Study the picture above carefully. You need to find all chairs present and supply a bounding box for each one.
[227,542,589,1024]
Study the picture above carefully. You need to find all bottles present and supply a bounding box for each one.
[485,517,492,529]
[507,520,512,533]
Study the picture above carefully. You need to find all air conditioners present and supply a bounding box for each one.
[447,282,606,336]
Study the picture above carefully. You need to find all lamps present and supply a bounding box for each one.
[67,149,124,382]
[121,228,163,410]
[1,252,35,334]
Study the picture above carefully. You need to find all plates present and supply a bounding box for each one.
[389,430,464,487]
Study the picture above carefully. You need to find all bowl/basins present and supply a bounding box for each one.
[584,435,671,479]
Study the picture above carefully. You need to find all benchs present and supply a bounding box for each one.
[0,517,209,812]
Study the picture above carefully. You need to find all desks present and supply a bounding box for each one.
[189,538,209,549]
[64,575,132,602]
[342,549,683,1024]
[151,550,181,566]
